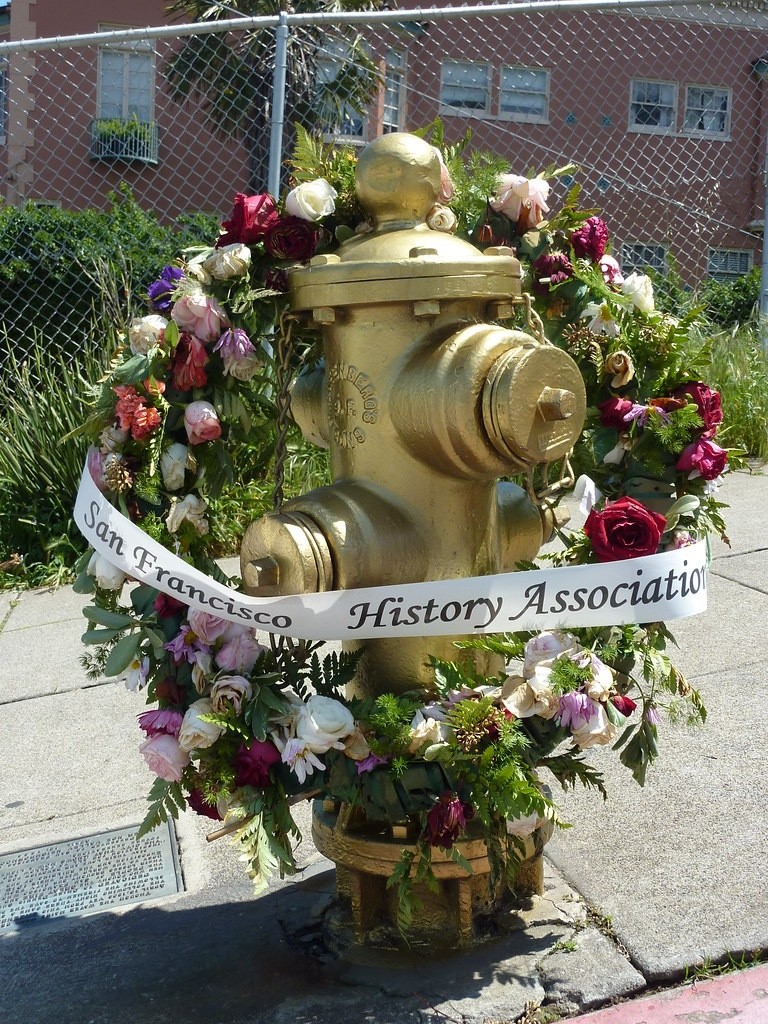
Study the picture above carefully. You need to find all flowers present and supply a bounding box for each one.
[58,116,752,946]
